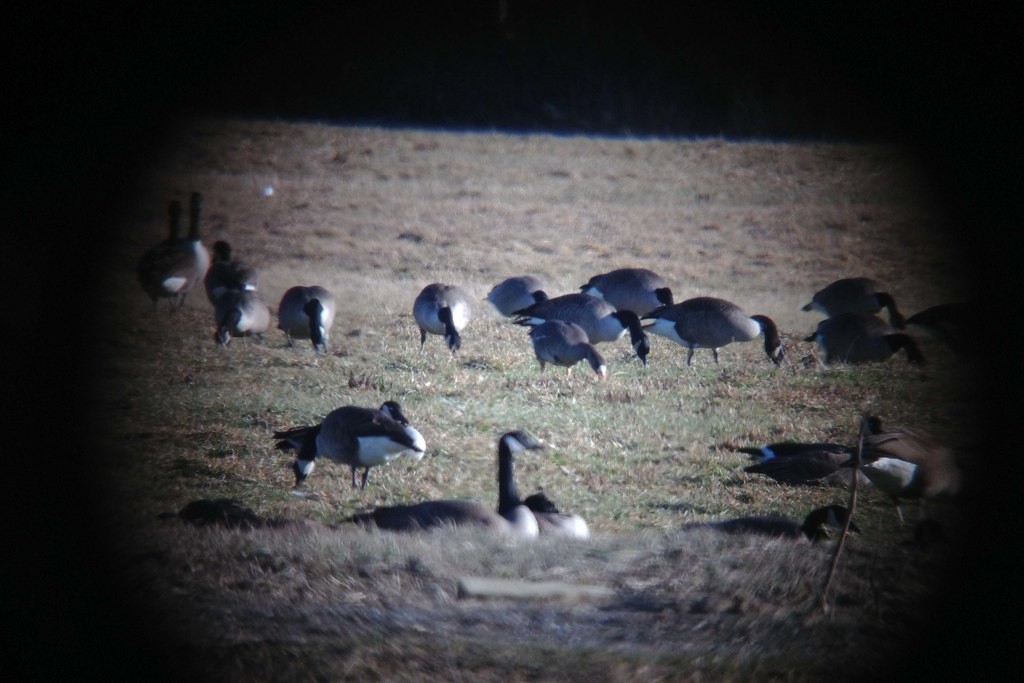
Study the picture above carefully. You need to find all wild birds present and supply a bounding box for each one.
[484,267,783,385]
[675,414,975,548]
[134,191,335,352]
[271,400,426,491]
[801,276,966,374]
[412,283,472,358]
[338,430,592,547]
